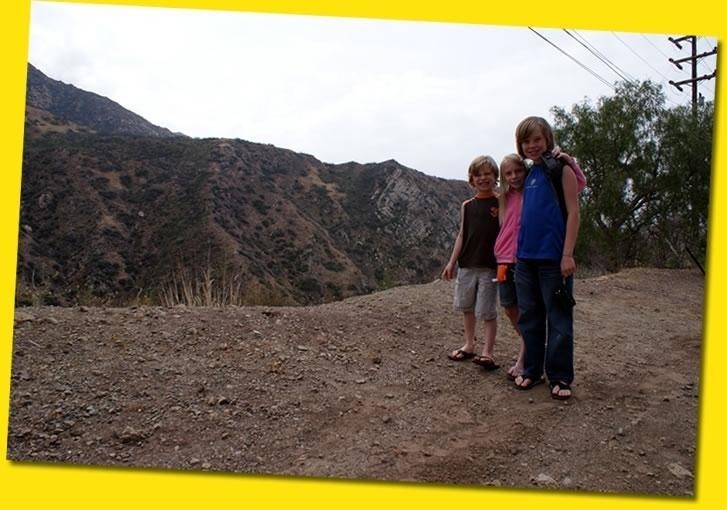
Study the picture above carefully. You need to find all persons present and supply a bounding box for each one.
[494,143,587,381]
[440,153,500,369]
[511,117,580,401]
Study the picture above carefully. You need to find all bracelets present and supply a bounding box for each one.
[562,254,572,258]
[448,261,454,265]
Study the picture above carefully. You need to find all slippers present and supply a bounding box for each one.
[448,349,476,361]
[549,380,571,400]
[472,356,500,369]
[507,366,545,390]
